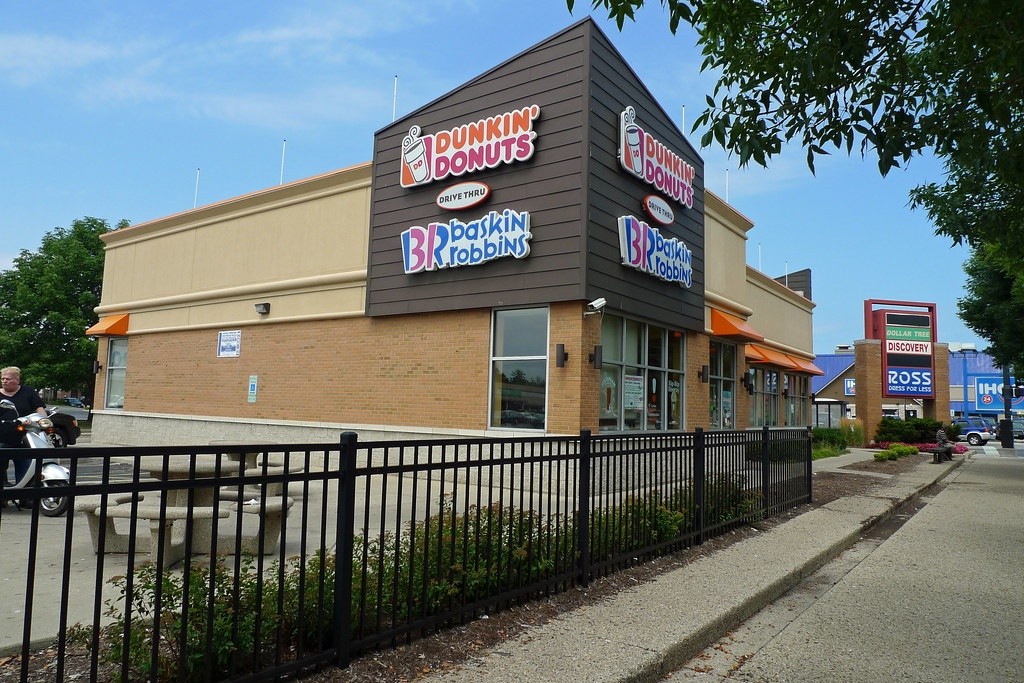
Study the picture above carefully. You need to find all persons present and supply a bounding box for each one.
[0,366,47,509]
[936,426,955,461]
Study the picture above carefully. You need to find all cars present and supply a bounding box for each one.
[1012,422,1024,440]
[984,417,997,432]
[63,397,85,408]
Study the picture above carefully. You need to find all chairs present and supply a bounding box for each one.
[73,439,305,570]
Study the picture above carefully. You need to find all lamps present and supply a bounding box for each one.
[254,302,270,315]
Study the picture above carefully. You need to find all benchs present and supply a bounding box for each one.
[928,446,952,465]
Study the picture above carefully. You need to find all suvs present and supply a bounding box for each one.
[951,418,996,446]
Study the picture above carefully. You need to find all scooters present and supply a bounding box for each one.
[0,399,71,517]
